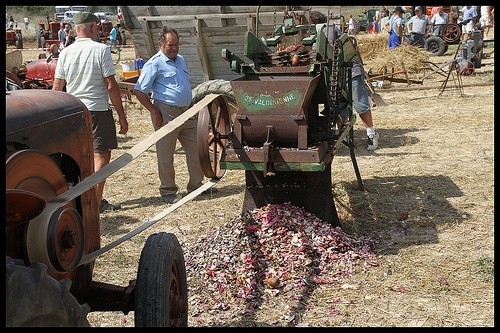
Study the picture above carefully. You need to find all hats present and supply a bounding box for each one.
[72,11,100,25]
[414,6,423,12]
[391,7,406,14]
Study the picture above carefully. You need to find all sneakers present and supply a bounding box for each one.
[367,132,380,151]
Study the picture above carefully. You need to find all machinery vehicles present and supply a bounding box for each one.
[5,4,490,328]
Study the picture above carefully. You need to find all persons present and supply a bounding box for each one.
[117,9,123,23]
[6,11,18,32]
[406,6,433,50]
[379,6,493,42]
[58,24,77,53]
[383,6,406,49]
[109,24,118,50]
[98,14,101,24]
[308,9,379,151]
[372,16,379,35]
[24,16,29,30]
[46,44,61,64]
[366,21,374,34]
[52,11,128,215]
[133,25,217,203]
[346,13,357,38]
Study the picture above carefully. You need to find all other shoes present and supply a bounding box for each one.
[162,194,180,204]
[188,184,218,194]
[99,199,121,213]
